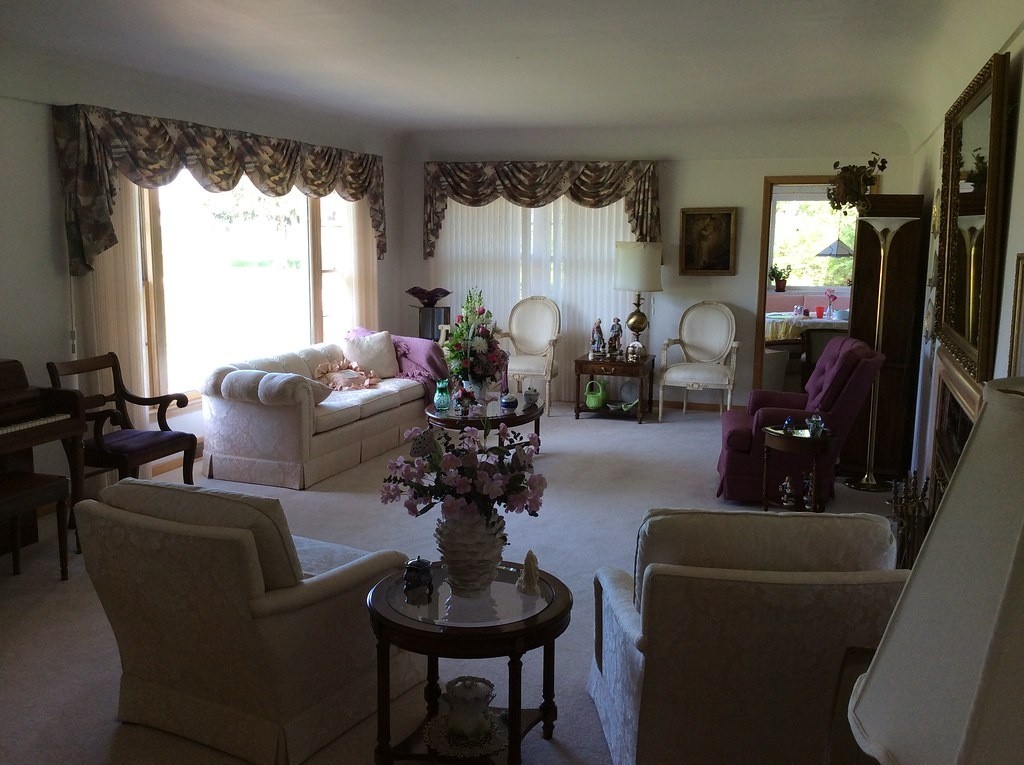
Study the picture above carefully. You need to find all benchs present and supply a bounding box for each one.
[766,293,849,313]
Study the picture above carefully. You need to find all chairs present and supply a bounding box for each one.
[491,295,562,417]
[46,353,197,530]
[658,300,740,422]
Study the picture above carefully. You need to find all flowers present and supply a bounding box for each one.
[379,288,547,548]
[824,288,838,304]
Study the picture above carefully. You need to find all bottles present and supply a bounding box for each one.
[792,304,809,317]
[501,394,518,412]
[401,555,434,605]
[434,381,450,410]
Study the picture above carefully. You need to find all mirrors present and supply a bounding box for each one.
[932,50,1011,383]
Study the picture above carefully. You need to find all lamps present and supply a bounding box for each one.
[848,377,1024,765]
[814,212,854,257]
[842,217,920,492]
[417,307,450,341]
[958,214,984,343]
[613,241,663,357]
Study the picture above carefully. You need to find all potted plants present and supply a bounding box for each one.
[768,264,792,292]
[827,148,925,217]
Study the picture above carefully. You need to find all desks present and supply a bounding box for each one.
[761,423,833,512]
[764,311,849,341]
[366,559,574,765]
[573,354,656,424]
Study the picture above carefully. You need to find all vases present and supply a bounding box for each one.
[826,303,834,319]
[461,380,488,399]
[434,507,509,598]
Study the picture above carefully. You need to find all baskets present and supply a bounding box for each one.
[584,380,602,409]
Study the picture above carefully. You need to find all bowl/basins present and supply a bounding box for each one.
[834,309,849,320]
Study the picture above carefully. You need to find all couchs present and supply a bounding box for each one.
[716,335,885,505]
[589,508,912,765]
[73,477,430,765]
[200,330,451,490]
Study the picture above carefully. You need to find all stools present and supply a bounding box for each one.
[0,468,69,581]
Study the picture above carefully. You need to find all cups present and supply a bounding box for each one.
[523,387,539,404]
[816,306,825,318]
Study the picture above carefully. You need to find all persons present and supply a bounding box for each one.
[590,318,603,352]
[610,318,622,350]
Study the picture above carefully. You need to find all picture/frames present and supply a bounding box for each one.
[679,207,737,275]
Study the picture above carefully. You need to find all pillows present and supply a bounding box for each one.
[307,378,334,405]
[315,359,382,393]
[342,330,399,379]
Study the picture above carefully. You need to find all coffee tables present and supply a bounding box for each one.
[426,391,546,464]
